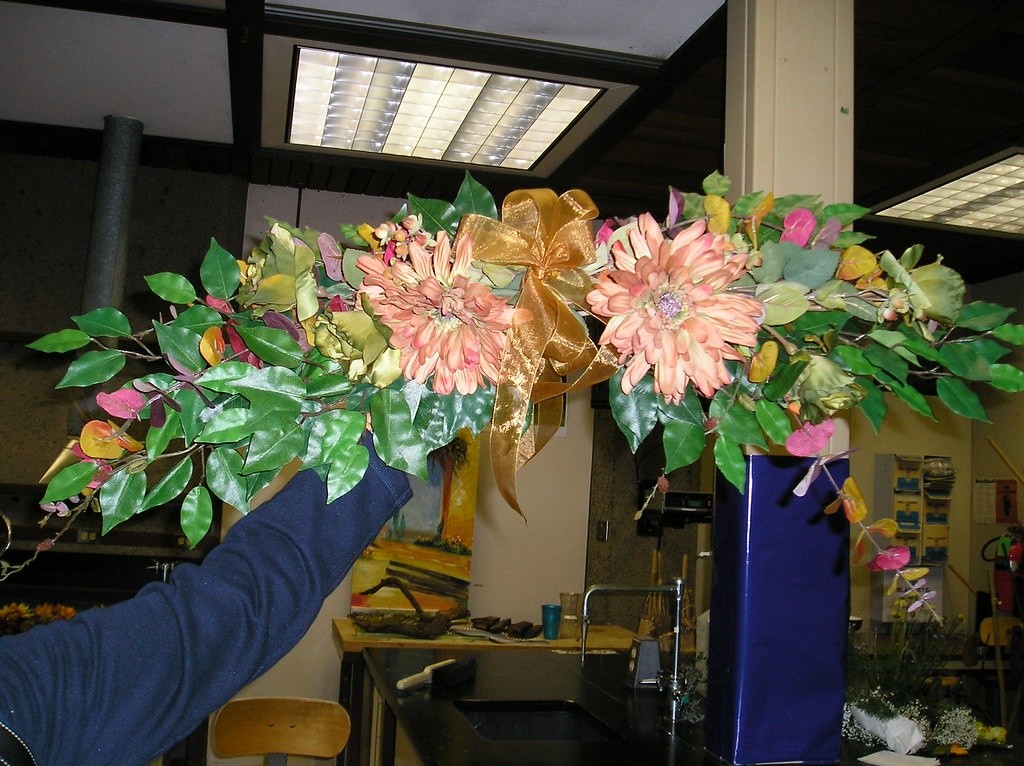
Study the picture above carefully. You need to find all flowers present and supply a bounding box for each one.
[0,169,1023,626]
[842,599,1013,758]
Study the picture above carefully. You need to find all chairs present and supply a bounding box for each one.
[211,696,352,766]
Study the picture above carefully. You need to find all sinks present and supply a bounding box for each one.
[453,699,628,744]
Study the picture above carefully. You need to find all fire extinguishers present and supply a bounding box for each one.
[980,525,1024,613]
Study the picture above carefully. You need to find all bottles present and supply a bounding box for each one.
[558,592,580,638]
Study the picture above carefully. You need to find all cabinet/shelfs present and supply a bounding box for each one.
[872,452,953,624]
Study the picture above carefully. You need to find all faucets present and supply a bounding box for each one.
[579,575,687,721]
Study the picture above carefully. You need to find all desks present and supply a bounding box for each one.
[331,619,711,766]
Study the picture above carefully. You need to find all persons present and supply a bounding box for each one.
[1,425,416,766]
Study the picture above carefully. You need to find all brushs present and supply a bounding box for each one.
[396,658,468,690]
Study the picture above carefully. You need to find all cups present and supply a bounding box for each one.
[542,604,562,640]
